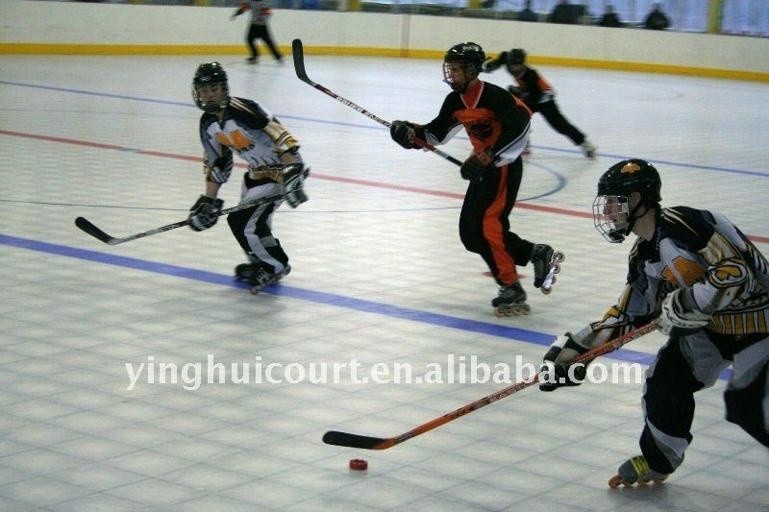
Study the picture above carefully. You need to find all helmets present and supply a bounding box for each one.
[442,41,485,92]
[190,61,228,111]
[508,48,525,75]
[592,158,661,243]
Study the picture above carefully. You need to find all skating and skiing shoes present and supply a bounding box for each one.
[249,264,291,295]
[493,281,531,318]
[530,243,565,294]
[609,455,668,489]
[236,263,255,283]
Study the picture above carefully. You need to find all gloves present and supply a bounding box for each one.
[461,145,496,179]
[282,163,308,208]
[391,119,426,149]
[656,287,710,337]
[538,331,593,392]
[188,195,223,232]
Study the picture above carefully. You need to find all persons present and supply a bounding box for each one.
[391,43,565,316]
[485,49,597,159]
[540,160,769,487]
[229,1,284,61]
[188,62,309,293]
[518,0,672,31]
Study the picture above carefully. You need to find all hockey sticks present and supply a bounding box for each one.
[323,318,661,449]
[75,194,286,246]
[293,38,465,167]
